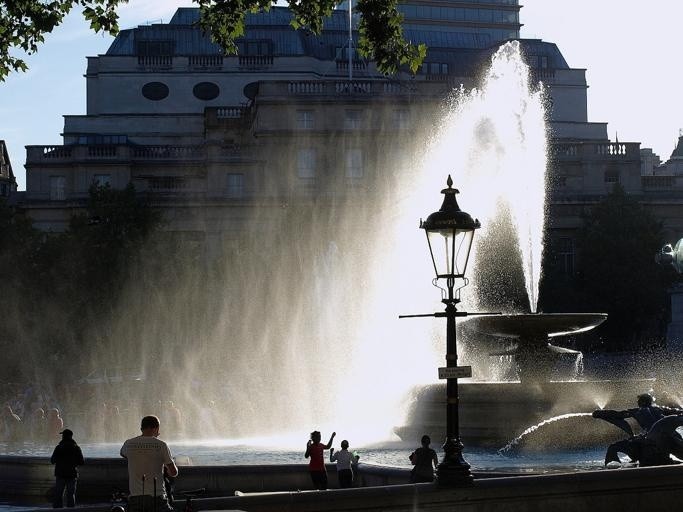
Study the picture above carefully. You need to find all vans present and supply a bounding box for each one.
[51,363,125,389]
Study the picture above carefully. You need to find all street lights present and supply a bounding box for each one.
[398,175,501,490]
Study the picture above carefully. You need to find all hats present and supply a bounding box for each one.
[60,429,73,435]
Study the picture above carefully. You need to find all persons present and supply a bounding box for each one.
[304,431,336,490]
[50,429,84,509]
[329,440,359,488]
[120,416,178,512]
[590,393,682,462]
[1,383,216,443]
[350,455,359,485]
[408,435,437,483]
